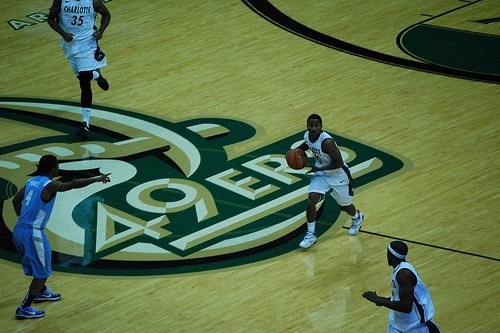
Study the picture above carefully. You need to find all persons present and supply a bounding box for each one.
[48,0,111,139]
[13,155,111,319]
[362,241,441,333]
[285,114,365,249]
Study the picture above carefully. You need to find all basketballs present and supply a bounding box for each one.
[286,149,307,170]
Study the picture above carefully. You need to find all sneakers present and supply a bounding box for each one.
[16,305,45,318]
[95,69,109,91]
[298,232,317,248]
[34,286,61,303]
[348,210,364,234]
[76,121,90,139]
